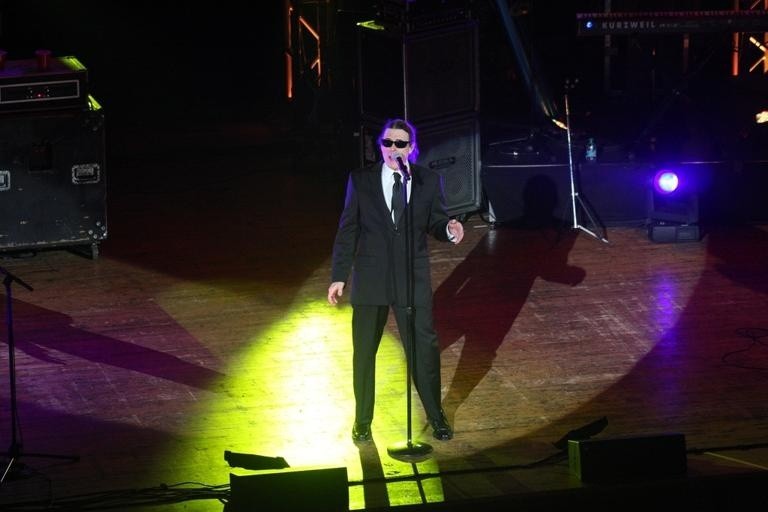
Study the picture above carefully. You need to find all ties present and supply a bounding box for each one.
[390,172,407,225]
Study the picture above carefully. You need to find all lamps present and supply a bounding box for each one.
[647,166,701,225]
[551,111,568,130]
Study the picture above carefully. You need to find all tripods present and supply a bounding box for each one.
[0,265,80,487]
[556,98,612,245]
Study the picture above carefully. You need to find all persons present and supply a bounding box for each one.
[326,117,466,442]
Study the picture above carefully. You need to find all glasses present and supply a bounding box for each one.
[379,137,410,149]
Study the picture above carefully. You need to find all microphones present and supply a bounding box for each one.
[393,152,411,180]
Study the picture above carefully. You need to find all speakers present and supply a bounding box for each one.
[568,431,688,483]
[359,117,481,218]
[357,18,480,125]
[230,463,349,512]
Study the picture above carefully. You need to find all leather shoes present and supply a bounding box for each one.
[351,422,373,441]
[428,412,453,440]
